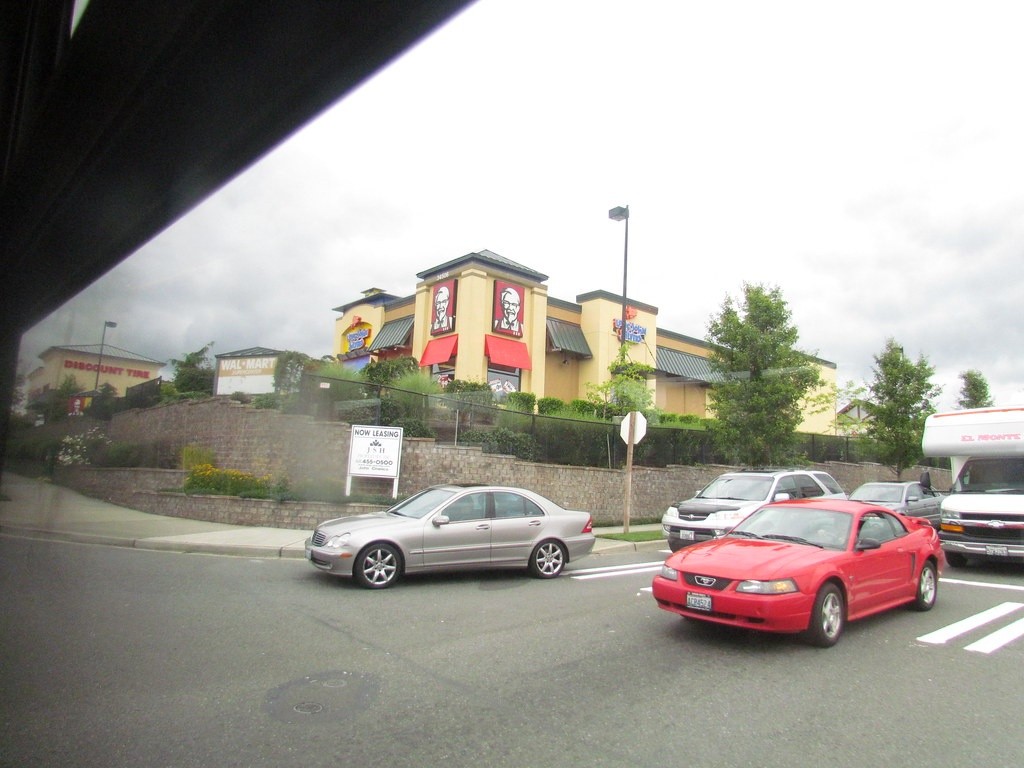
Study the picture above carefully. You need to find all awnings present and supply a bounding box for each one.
[655,344,730,389]
[366,311,415,356]
[484,334,532,372]
[544,315,593,360]
[25,394,52,411]
[418,332,458,368]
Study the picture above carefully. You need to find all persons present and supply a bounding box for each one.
[816,514,850,545]
[458,499,476,521]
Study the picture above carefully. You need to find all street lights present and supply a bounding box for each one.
[94,321,117,390]
[608,204,629,362]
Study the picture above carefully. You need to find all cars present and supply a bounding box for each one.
[847,480,948,531]
[652,498,946,648]
[304,483,596,589]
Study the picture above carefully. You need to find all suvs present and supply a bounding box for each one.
[660,466,849,555]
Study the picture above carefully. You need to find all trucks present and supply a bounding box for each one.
[921,405,1024,568]
